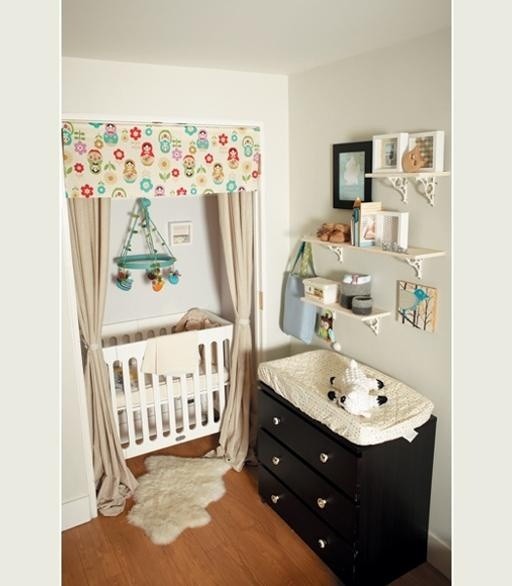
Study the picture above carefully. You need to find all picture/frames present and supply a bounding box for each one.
[331,139,373,210]
[372,129,445,173]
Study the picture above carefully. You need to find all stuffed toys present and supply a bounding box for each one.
[328,360,387,418]
[174,307,221,365]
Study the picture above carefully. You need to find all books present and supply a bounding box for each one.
[353,200,382,248]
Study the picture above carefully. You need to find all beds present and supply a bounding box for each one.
[79,308,236,465]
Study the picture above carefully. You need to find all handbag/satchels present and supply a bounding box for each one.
[283,240,319,346]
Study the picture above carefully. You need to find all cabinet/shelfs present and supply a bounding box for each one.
[255,380,437,586]
[299,172,450,336]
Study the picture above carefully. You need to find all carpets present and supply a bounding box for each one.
[126,453,231,547]
